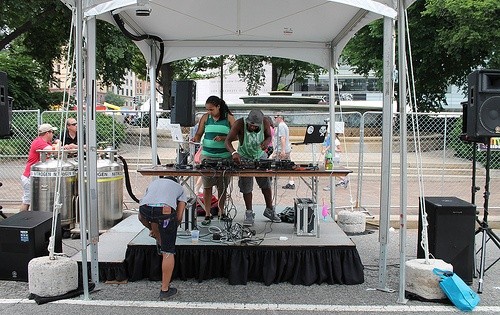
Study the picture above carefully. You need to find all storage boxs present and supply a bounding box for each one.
[293,198,317,236]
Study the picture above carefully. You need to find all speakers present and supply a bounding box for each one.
[459,102,488,144]
[466,69,500,137]
[170,80,196,126]
[419,196,476,285]
[0,211,63,283]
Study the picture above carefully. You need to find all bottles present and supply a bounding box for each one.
[325,150,332,170]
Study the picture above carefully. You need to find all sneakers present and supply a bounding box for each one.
[157,242,162,256]
[243,213,255,227]
[160,287,178,301]
[263,207,281,223]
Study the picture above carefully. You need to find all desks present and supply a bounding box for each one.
[137,165,353,231]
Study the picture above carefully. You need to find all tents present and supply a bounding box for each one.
[60,0,415,304]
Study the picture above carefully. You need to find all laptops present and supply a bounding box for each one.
[291,124,328,144]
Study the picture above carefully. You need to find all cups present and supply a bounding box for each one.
[190,230,199,242]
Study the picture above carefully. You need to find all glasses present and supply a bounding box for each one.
[70,123,77,126]
[47,131,54,134]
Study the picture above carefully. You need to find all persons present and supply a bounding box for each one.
[266,117,274,181]
[138,176,187,301]
[55,118,87,160]
[225,109,281,226]
[190,96,239,225]
[21,124,62,212]
[272,114,295,189]
[322,134,348,190]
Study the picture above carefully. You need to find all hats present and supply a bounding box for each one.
[246,109,263,127]
[38,124,57,134]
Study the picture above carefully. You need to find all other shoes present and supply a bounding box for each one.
[202,218,211,225]
[344,179,349,188]
[282,184,295,189]
[199,187,203,194]
[323,186,331,191]
[218,214,233,222]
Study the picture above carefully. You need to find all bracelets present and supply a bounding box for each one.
[231,151,239,155]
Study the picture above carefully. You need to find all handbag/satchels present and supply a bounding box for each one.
[276,205,295,224]
[196,193,220,217]
[194,147,202,165]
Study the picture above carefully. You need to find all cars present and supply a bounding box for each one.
[51,100,135,116]
[124,112,159,128]
